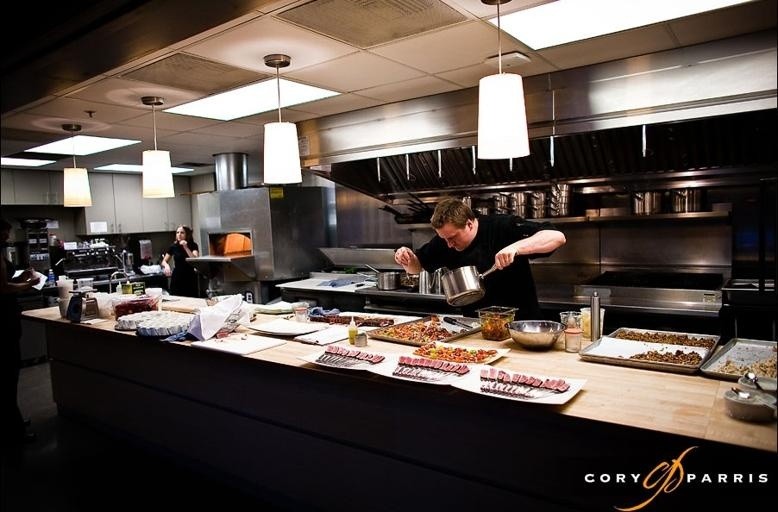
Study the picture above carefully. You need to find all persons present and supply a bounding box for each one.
[394,198,567,320]
[0,221,38,452]
[161,226,199,298]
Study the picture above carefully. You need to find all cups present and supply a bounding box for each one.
[355,334,367,346]
[560,311,583,353]
[56,280,74,318]
[293,306,310,322]
[580,307,606,338]
[76,277,94,289]
[585,207,628,218]
[122,284,132,294]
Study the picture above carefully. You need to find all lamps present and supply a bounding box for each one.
[62,124,93,207]
[261,54,304,186]
[476,1,532,161]
[141,96,176,199]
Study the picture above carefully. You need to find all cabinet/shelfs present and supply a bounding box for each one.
[14,229,52,272]
[141,175,193,232]
[12,169,64,206]
[0,168,15,205]
[75,172,143,235]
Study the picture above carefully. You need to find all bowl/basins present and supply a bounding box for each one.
[504,320,566,350]
[724,376,777,422]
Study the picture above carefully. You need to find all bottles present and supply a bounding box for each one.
[48,269,55,287]
[590,290,601,340]
[347,315,358,345]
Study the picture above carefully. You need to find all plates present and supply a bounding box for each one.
[294,346,587,405]
[413,339,511,364]
[239,317,322,336]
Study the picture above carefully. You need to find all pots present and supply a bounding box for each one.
[379,183,574,223]
[440,262,501,305]
[418,269,438,295]
[357,263,402,290]
[634,187,708,216]
